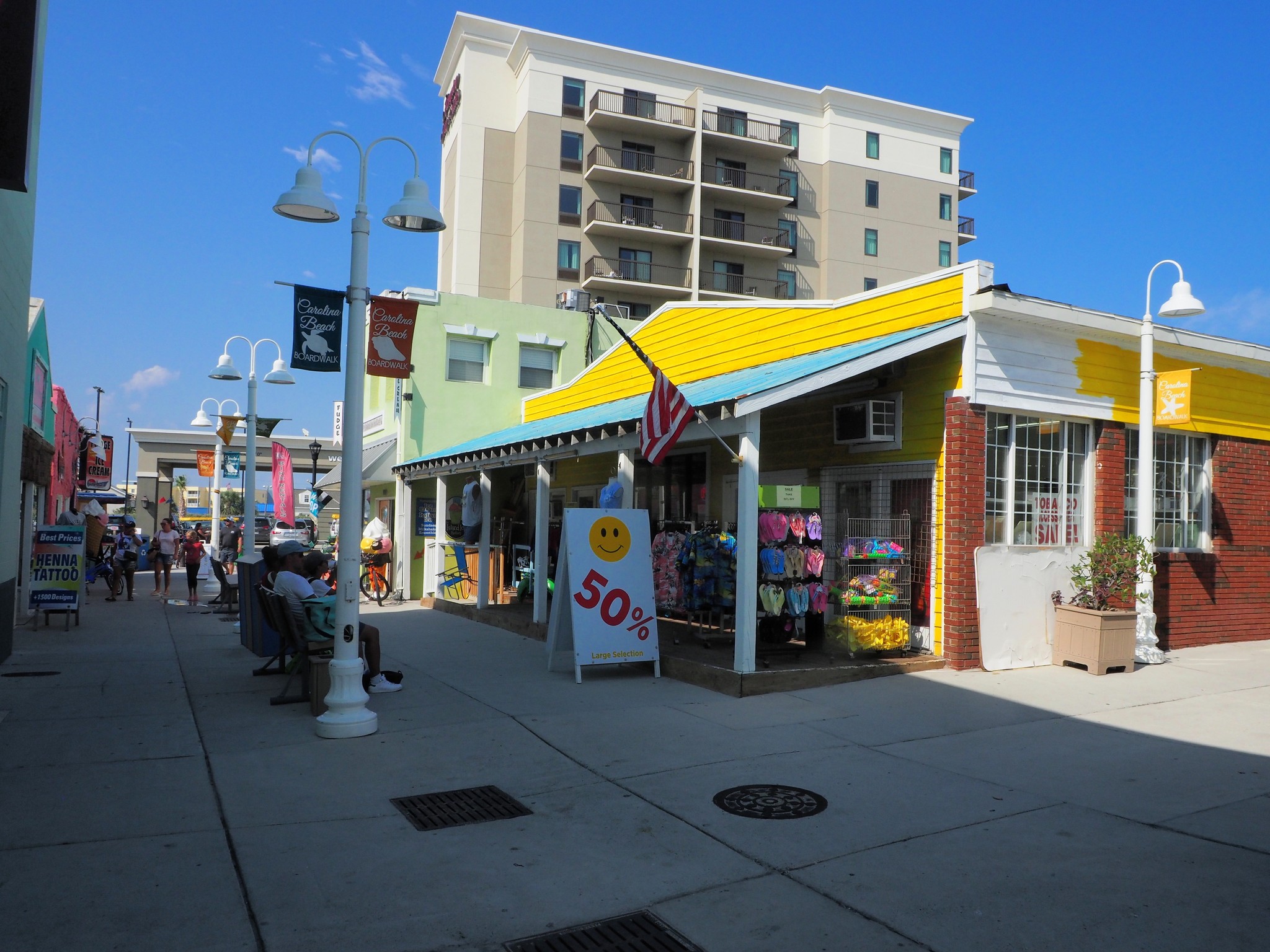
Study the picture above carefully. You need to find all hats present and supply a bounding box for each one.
[277,540,311,557]
[304,552,332,561]
[123,521,136,528]
[223,516,234,522]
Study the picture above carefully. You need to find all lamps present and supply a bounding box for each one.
[141,496,148,508]
[397,474,407,480]
[414,450,578,478]
[62,416,107,462]
[129,496,136,508]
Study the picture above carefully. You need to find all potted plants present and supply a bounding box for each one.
[1051,531,1160,676]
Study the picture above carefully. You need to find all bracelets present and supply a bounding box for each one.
[110,556,113,558]
[132,535,136,538]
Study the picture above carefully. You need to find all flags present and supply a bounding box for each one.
[1154,368,1192,426]
[216,415,245,447]
[364,295,419,379]
[608,313,694,467]
[223,452,241,478]
[255,418,282,439]
[309,484,332,518]
[159,497,179,511]
[197,450,215,477]
[226,482,231,490]
[272,441,295,528]
[290,282,346,372]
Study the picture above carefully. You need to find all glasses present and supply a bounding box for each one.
[160,522,171,526]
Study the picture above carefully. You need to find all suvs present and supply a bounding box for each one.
[302,518,318,544]
[328,519,339,538]
[230,515,244,527]
[238,516,274,544]
[200,519,227,545]
[170,513,193,544]
[106,514,135,536]
[269,518,310,548]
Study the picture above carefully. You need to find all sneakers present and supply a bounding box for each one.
[517,555,530,568]
[368,674,403,694]
[363,669,370,675]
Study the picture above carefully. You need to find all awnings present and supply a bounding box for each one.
[313,439,396,490]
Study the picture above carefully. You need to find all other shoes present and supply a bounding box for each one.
[127,596,134,601]
[226,572,229,575]
[105,596,117,601]
[187,595,199,601]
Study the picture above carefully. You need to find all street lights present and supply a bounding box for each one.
[1133,259,1206,663]
[93,386,105,493]
[272,128,447,739]
[308,437,322,549]
[207,336,296,633]
[190,398,247,592]
[124,420,133,515]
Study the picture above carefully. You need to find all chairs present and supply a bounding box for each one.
[669,168,683,177]
[746,286,757,296]
[761,237,772,246]
[612,269,623,279]
[754,186,765,192]
[722,178,733,187]
[641,164,655,174]
[594,268,604,276]
[648,113,661,121]
[435,543,479,600]
[622,213,663,229]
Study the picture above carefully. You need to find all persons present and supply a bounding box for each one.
[194,523,211,543]
[461,474,483,545]
[57,508,87,526]
[85,514,107,559]
[219,516,243,575]
[150,518,179,596]
[261,540,402,693]
[599,477,625,509]
[105,521,142,601]
[176,531,206,602]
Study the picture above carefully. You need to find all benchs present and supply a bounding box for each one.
[254,581,363,705]
[208,557,240,614]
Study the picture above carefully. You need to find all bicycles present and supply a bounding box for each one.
[85,545,124,596]
[360,539,390,607]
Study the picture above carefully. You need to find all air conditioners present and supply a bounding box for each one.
[594,303,630,319]
[833,400,895,445]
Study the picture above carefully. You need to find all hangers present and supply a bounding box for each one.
[703,520,722,535]
[665,519,686,535]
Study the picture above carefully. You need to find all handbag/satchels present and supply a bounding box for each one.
[146,545,161,562]
[362,670,403,692]
[124,550,137,561]
[300,594,334,643]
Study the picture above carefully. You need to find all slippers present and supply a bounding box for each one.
[783,548,804,578]
[805,583,827,612]
[151,591,162,596]
[805,514,823,541]
[165,592,170,596]
[798,547,824,577]
[760,547,785,575]
[759,584,785,615]
[759,511,790,543]
[786,587,809,617]
[789,513,806,538]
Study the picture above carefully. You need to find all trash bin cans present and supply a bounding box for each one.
[235,554,296,657]
[135,534,150,570]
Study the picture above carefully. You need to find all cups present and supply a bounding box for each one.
[327,559,338,568]
